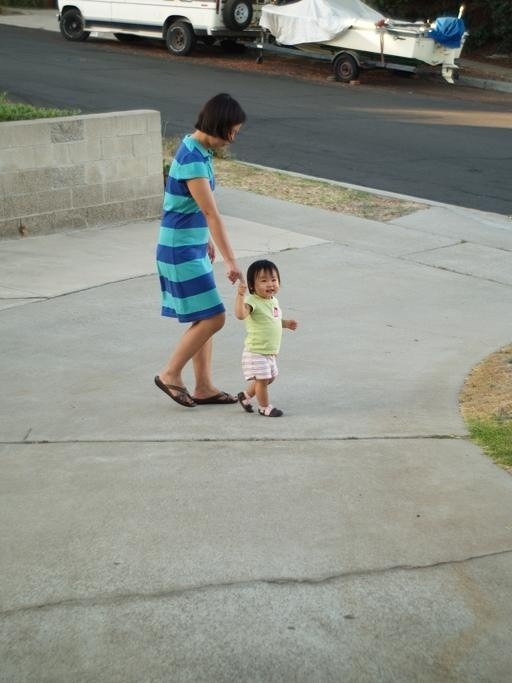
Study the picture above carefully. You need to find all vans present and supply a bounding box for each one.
[57,0,275,55]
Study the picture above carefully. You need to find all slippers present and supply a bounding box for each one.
[154,375,196,408]
[191,391,240,404]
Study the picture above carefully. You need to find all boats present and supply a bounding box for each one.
[262,4,468,85]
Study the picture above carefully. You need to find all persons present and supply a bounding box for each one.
[235,259,298,417]
[153,91,248,408]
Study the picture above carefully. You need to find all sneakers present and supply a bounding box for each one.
[258,404,282,417]
[238,392,254,413]
